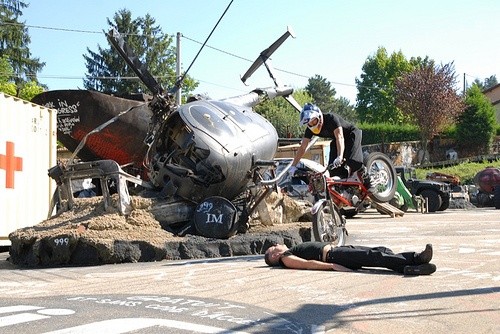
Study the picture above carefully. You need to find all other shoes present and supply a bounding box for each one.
[417,244,432,265]
[404,264,436,275]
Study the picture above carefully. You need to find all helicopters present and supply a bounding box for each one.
[101,25,304,230]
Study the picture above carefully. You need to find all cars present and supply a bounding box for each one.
[262,158,371,219]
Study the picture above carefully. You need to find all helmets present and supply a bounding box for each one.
[299,104,324,134]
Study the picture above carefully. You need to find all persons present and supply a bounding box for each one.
[289,104,368,181]
[264,240,436,275]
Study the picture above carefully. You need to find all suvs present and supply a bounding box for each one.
[396,165,451,213]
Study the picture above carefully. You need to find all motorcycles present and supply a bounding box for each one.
[294,152,397,246]
